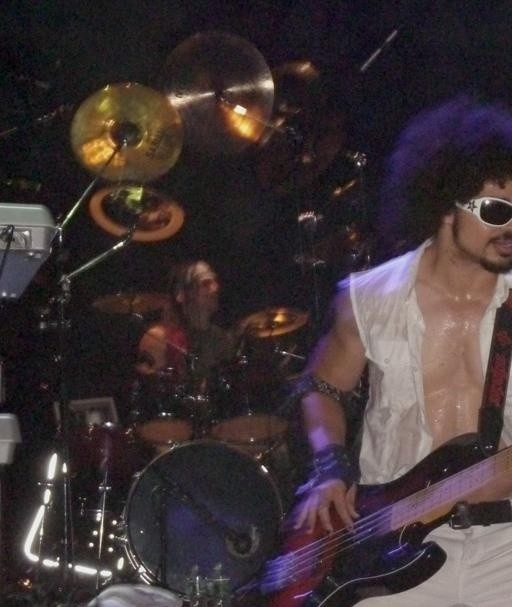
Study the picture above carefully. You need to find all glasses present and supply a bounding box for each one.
[455,196,511,227]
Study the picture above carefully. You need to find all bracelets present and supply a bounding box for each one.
[302,444,353,487]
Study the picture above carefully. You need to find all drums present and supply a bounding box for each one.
[51,422,144,522]
[199,399,293,462]
[129,408,199,465]
[124,438,290,605]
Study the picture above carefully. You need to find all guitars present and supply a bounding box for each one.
[250,434,512,607]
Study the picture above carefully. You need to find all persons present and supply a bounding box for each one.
[289,124,512,607]
[126,257,226,458]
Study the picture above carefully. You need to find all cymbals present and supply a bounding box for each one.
[88,185,185,243]
[234,333,317,413]
[162,29,276,168]
[238,305,310,339]
[72,82,184,186]
[90,291,172,316]
[254,59,328,189]
